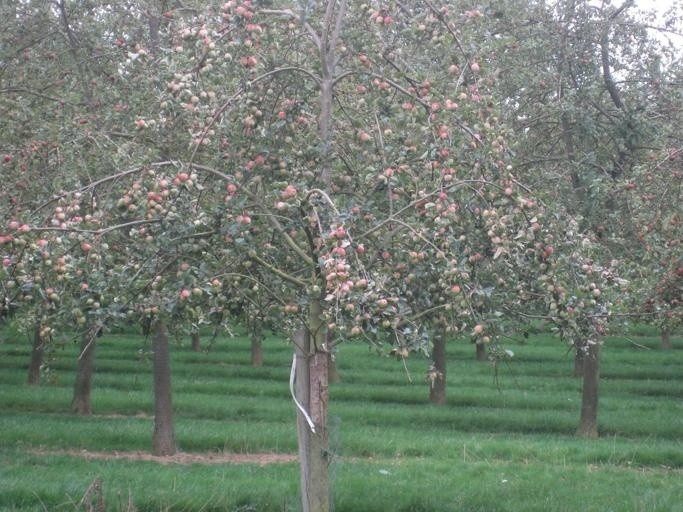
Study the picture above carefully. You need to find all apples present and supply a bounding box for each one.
[0,0,683,358]
